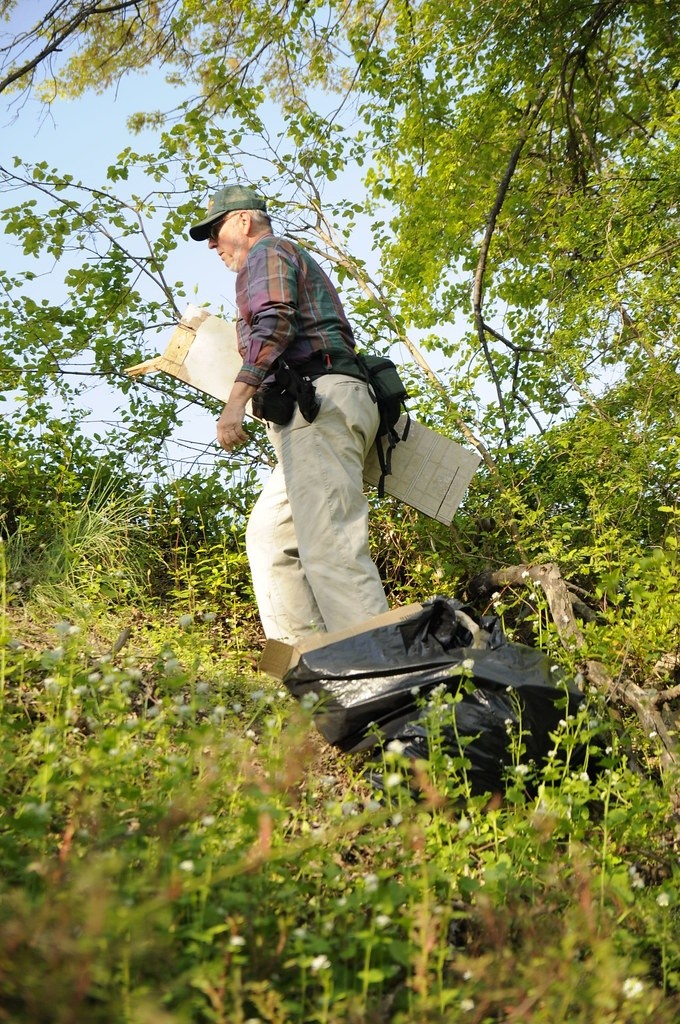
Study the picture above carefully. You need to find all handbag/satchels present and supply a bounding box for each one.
[305,348,409,438]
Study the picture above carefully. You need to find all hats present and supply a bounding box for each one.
[188,184,267,242]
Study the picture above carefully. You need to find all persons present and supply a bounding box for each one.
[190,186,391,648]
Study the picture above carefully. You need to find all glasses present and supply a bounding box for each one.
[207,211,241,241]
